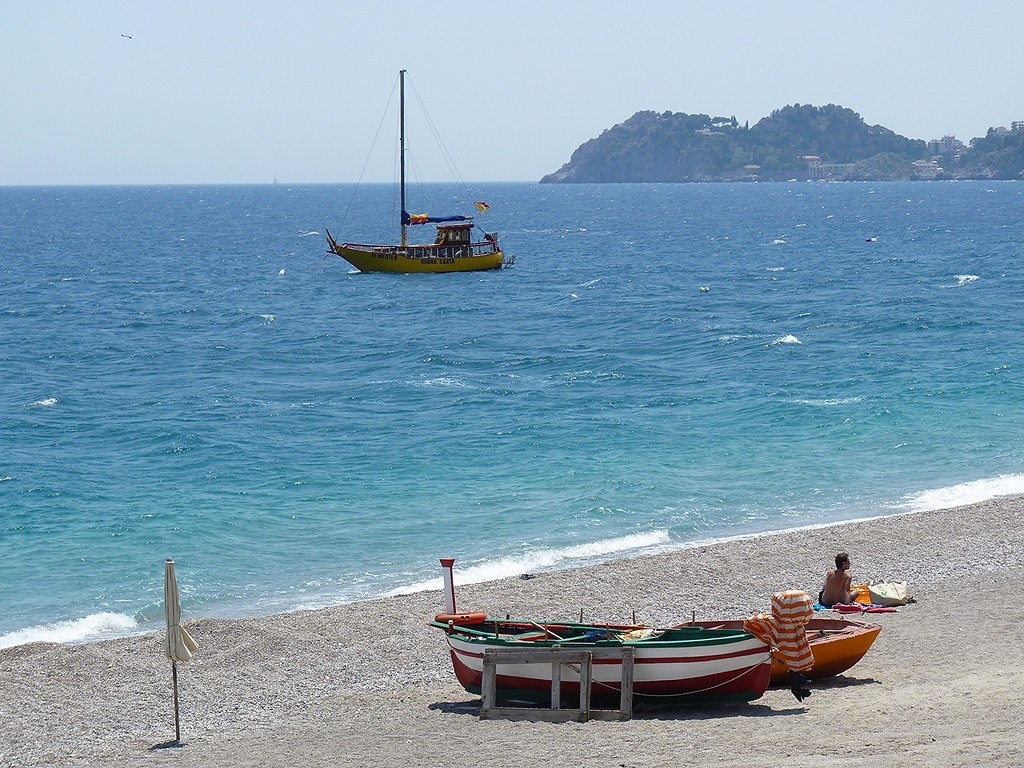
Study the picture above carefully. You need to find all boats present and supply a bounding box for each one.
[428,555,880,706]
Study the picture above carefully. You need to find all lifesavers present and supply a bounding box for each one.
[435,610,486,626]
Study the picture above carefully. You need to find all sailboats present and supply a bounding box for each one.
[323,68,517,271]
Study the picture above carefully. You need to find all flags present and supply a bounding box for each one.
[474,201,490,213]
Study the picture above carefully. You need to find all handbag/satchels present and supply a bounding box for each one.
[867,575,911,607]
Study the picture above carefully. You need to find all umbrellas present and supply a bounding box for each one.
[165,558,199,740]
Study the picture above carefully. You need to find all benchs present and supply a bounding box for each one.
[554,634,590,641]
[509,632,551,639]
[624,634,664,642]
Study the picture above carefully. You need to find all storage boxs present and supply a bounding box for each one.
[850,585,872,604]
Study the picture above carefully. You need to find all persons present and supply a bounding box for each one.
[818,553,859,607]
